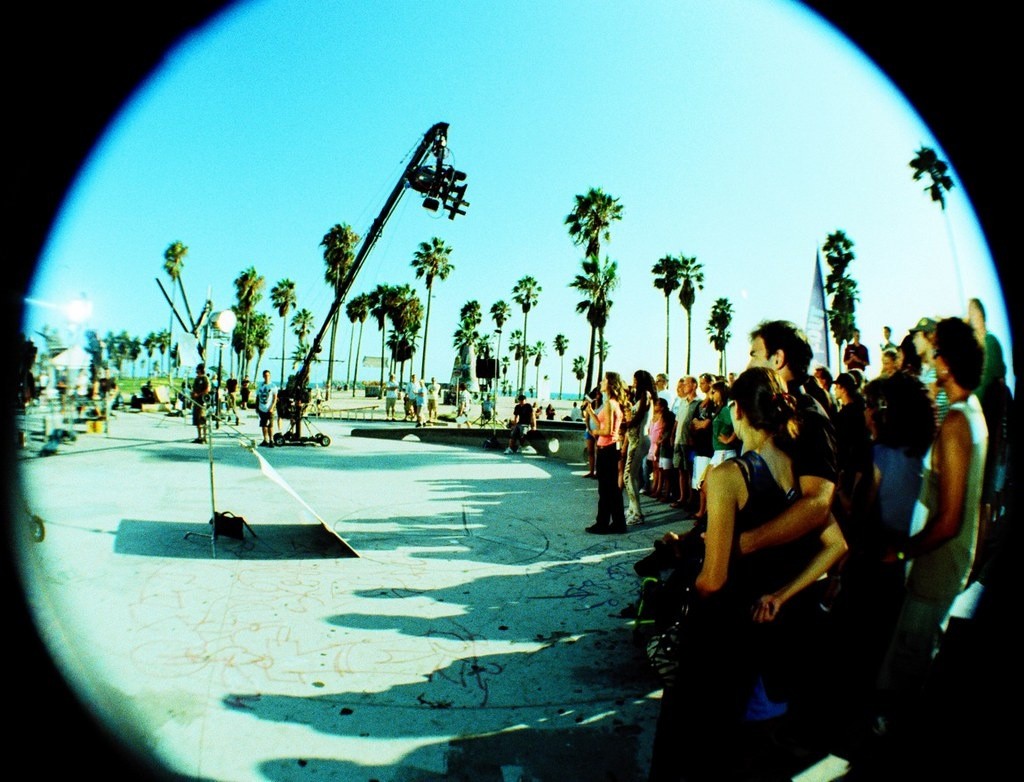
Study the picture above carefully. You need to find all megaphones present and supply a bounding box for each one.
[202,394,212,404]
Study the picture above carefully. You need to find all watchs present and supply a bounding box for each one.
[894,544,907,562]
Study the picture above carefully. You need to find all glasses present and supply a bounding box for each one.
[929,348,939,360]
[711,389,717,395]
[656,379,666,382]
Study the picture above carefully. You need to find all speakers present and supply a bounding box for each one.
[476,359,499,378]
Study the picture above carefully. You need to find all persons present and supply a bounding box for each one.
[72,369,90,413]
[621,370,658,525]
[504,395,537,454]
[650,367,848,782]
[872,316,990,735]
[179,374,250,410]
[384,374,399,421]
[581,371,626,534]
[473,298,1014,782]
[427,378,441,423]
[100,367,117,419]
[255,371,278,448]
[192,364,210,444]
[404,375,419,420]
[413,380,427,427]
[457,382,472,428]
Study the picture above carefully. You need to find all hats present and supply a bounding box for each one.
[909,317,938,338]
[830,373,855,389]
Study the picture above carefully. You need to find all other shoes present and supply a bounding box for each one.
[584,473,594,478]
[269,440,274,447]
[642,490,653,496]
[259,440,268,445]
[585,522,609,535]
[516,446,522,453]
[609,522,628,534]
[192,438,207,444]
[690,513,703,520]
[504,447,514,455]
[660,497,672,503]
[650,492,662,498]
[626,513,645,525]
[624,509,634,518]
[670,501,686,509]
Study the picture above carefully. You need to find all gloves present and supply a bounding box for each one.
[646,585,697,674]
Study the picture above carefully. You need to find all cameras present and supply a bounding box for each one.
[581,405,586,410]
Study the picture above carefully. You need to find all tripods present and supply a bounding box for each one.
[183,405,254,558]
[472,379,505,429]
